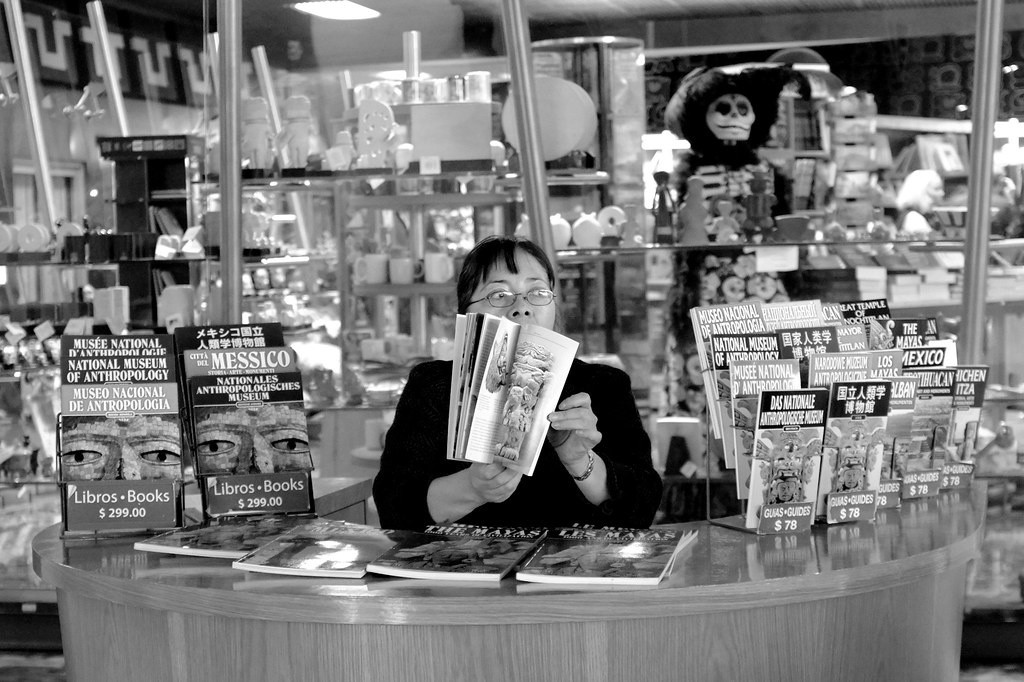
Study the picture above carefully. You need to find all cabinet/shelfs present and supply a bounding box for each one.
[203,162,644,396]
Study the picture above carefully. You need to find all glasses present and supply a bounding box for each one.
[464,289,556,310]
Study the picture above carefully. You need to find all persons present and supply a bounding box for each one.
[367,234,662,534]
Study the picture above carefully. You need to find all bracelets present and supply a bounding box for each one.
[570,451,595,480]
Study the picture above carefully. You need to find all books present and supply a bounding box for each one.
[60,322,316,537]
[686,297,990,535]
[446,312,580,476]
[128,517,700,588]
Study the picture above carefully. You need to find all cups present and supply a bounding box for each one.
[425,253,455,285]
[354,254,388,283]
[390,257,425,285]
[364,420,388,450]
